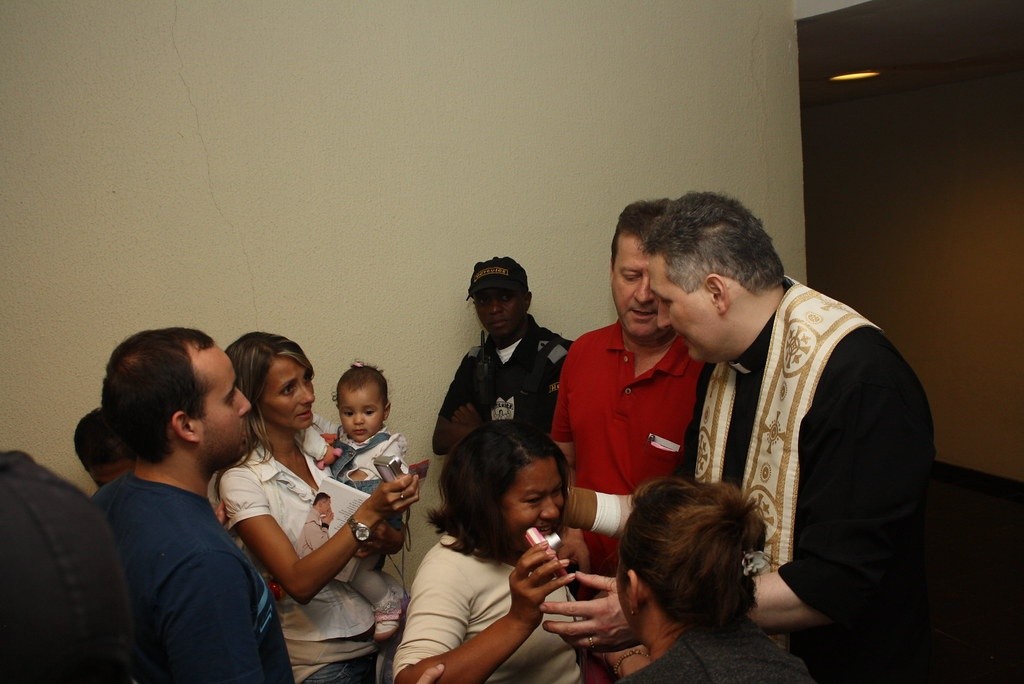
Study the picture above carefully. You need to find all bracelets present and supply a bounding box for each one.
[590,492,621,537]
[613,648,651,675]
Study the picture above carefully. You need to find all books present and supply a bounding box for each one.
[295,476,373,583]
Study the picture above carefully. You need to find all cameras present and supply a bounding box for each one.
[524,525,569,580]
[372,455,406,483]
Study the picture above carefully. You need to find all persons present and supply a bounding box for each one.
[73,406,135,500]
[392,423,587,684]
[90,326,296,684]
[216,332,420,684]
[295,491,334,559]
[0,449,139,684]
[549,196,706,684]
[433,256,567,468]
[330,361,412,641]
[539,191,940,684]
[614,476,814,684]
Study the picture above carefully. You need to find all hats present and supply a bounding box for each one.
[466,256,528,301]
[0,450,134,684]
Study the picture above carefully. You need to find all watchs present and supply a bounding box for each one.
[347,515,372,544]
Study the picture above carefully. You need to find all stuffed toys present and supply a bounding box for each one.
[315,432,343,470]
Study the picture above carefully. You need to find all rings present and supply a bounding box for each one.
[589,636,595,648]
[400,492,404,499]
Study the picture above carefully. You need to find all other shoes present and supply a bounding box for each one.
[373,588,409,641]
